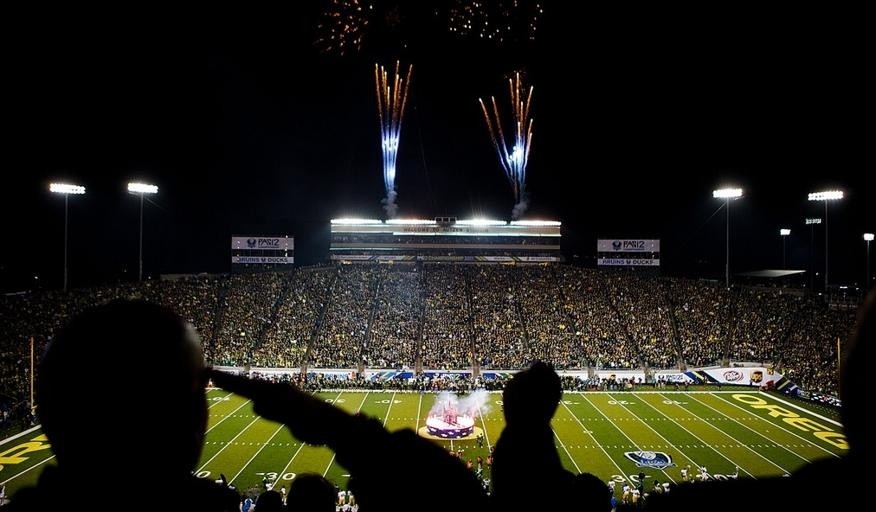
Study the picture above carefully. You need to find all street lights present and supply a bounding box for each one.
[805,218,823,241]
[45,178,88,290]
[779,227,793,268]
[863,233,874,258]
[710,183,747,289]
[807,187,846,285]
[124,179,163,279]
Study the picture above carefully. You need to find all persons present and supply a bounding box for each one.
[1,297,496,512]
[236,494,258,512]
[2,232,876,495]
[608,478,671,510]
[335,489,360,512]
[278,490,288,505]
[488,286,876,508]
